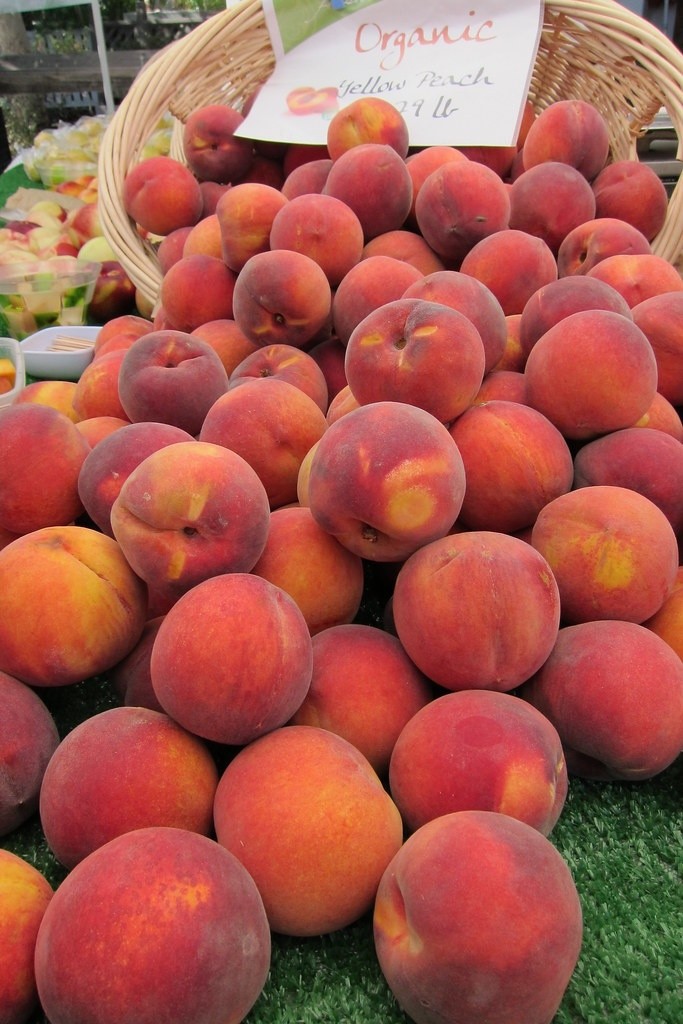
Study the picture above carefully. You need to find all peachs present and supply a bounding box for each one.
[0,84,682,1024]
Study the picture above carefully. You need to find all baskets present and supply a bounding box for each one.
[96,1,683,317]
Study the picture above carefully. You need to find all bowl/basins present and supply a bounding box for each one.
[0,256,103,321]
[19,326,104,380]
[0,336,26,412]
